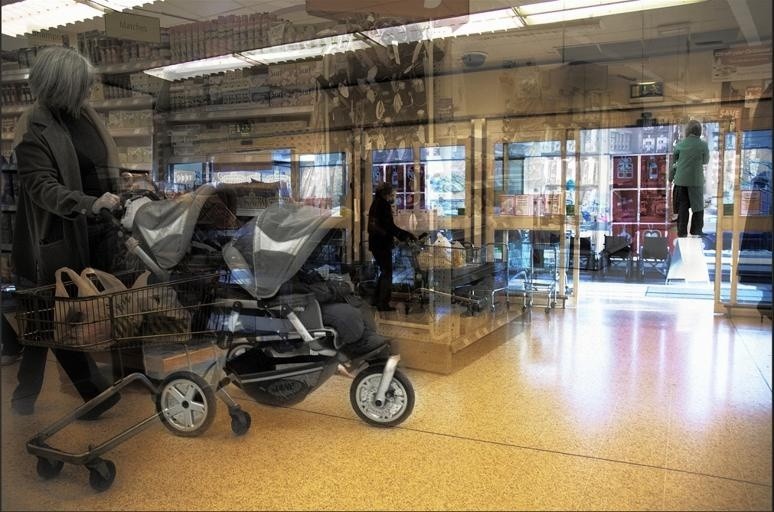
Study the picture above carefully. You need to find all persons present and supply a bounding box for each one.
[368,182,417,311]
[1,43,131,421]
[672,120,709,238]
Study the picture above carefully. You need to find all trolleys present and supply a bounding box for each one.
[14,265,250,491]
[406,238,487,318]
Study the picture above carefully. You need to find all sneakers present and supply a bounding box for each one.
[0,355,23,366]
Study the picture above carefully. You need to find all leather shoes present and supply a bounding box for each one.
[12,399,34,415]
[672,213,707,237]
[371,303,396,311]
[86,392,121,420]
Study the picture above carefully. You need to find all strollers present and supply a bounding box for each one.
[100,188,414,428]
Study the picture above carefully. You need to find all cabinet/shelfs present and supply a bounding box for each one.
[0,58,166,253]
